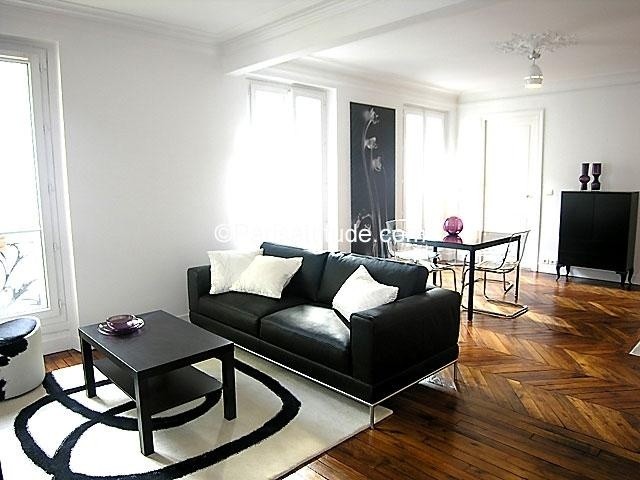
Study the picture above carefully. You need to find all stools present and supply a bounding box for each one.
[0,316,46,400]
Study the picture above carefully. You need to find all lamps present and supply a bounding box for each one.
[523,49,544,91]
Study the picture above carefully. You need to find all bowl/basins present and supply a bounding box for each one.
[106,315,134,330]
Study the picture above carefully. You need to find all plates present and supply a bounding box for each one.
[97,317,144,336]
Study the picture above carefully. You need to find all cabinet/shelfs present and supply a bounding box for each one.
[553,189,639,290]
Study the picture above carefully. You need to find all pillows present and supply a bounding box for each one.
[330,263,399,324]
[208,246,305,301]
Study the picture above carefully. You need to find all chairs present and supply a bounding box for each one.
[385,219,533,320]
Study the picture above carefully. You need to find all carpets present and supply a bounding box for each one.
[1,341,394,480]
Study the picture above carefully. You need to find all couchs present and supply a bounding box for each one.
[187,243,463,432]
